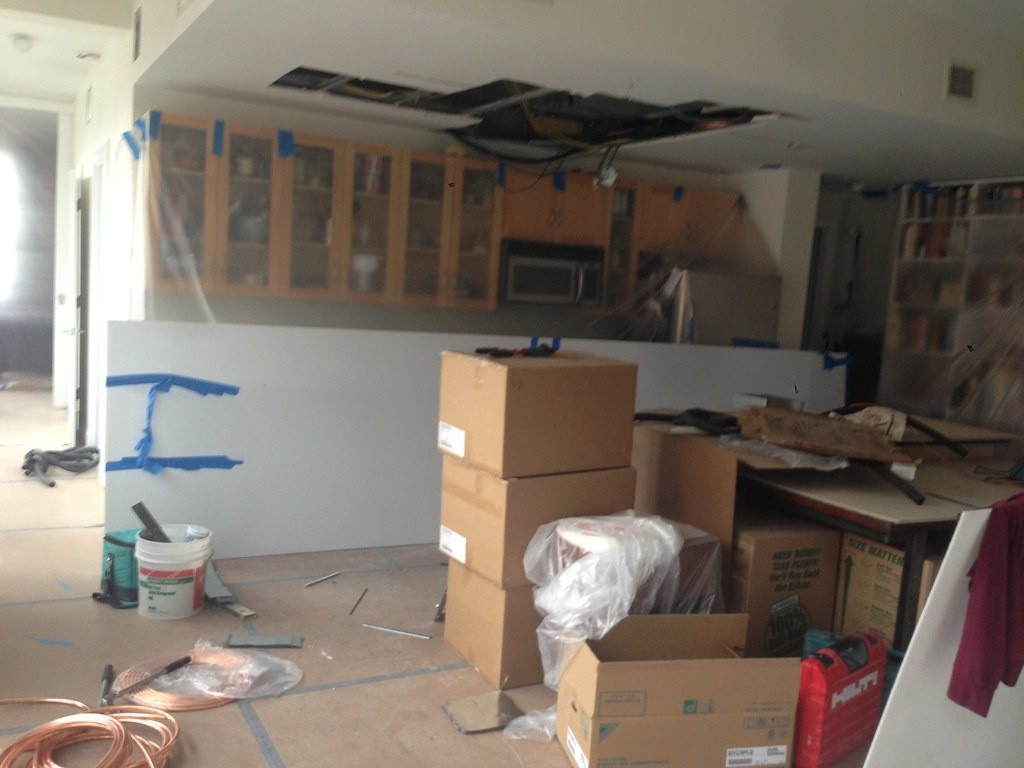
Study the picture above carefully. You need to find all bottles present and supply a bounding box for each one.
[899,185,1024,354]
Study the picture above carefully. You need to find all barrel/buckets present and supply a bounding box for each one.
[134,523,215,619]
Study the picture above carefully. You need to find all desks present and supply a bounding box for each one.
[739,464,982,650]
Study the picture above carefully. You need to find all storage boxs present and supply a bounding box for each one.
[437,349,946,768]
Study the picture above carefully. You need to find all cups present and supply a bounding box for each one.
[354,255,380,290]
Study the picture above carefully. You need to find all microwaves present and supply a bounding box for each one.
[498,238,605,308]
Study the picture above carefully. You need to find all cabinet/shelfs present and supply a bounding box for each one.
[876,176,1023,433]
[116,110,745,313]
